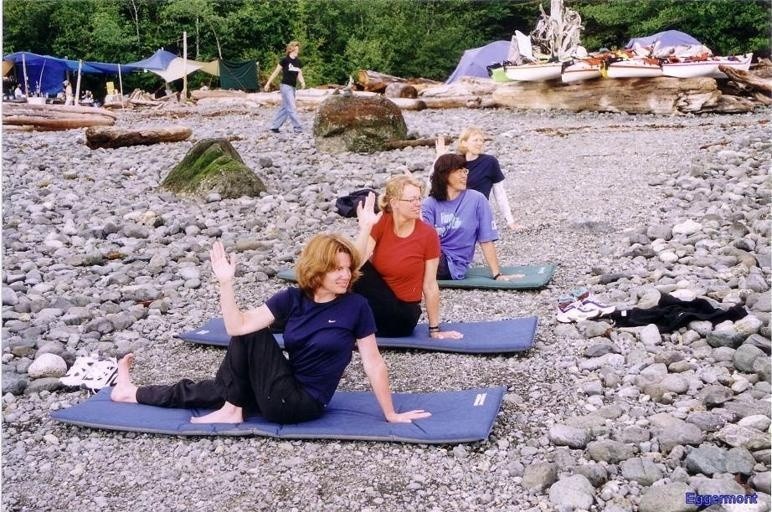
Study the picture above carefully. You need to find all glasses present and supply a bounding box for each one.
[456,168,470,176]
[399,196,423,205]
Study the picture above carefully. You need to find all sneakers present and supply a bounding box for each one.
[271,128,280,133]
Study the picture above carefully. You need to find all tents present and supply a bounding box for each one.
[444,40,512,86]
[624,29,710,60]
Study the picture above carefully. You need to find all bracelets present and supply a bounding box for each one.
[493,272,504,280]
[429,326,439,332]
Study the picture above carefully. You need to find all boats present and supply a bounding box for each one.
[503,49,754,84]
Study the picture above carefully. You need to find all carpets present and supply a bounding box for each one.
[275,262,560,293]
[172,316,537,353]
[50,384,509,447]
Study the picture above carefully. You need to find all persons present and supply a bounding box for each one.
[14,80,94,105]
[268,177,463,341]
[110,234,431,424]
[264,41,306,133]
[421,154,526,282]
[428,127,517,230]
